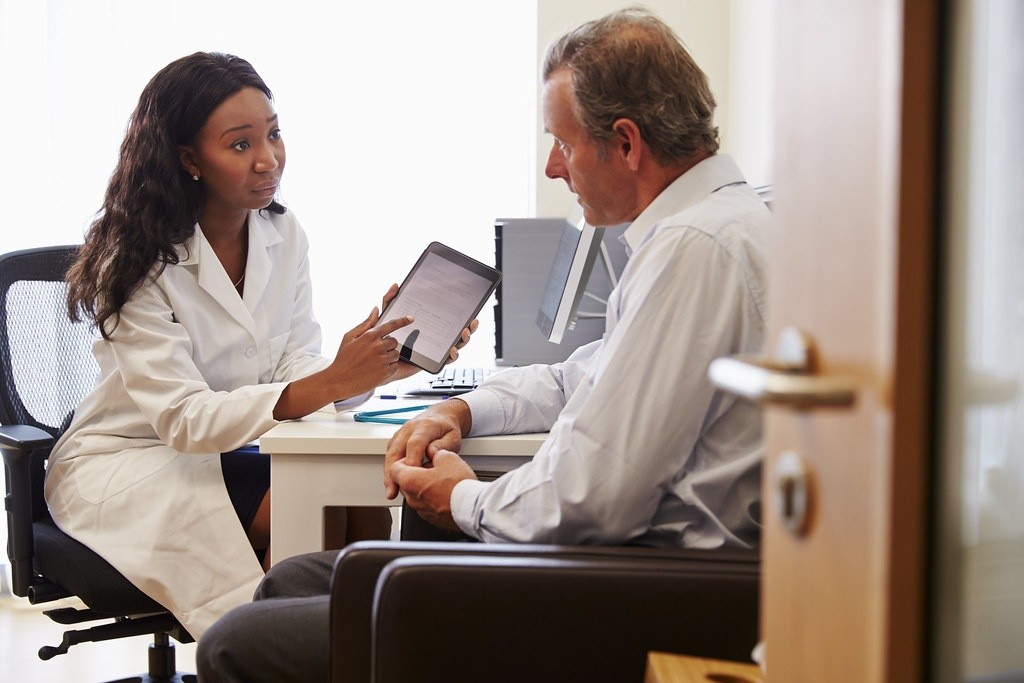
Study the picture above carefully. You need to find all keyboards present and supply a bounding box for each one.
[429,367,485,390]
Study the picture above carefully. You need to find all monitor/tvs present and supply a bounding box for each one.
[537,210,606,344]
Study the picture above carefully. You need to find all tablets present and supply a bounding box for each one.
[373,240,502,374]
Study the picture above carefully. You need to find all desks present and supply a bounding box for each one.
[257,399,552,570]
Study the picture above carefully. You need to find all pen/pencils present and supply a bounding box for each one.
[372,394,448,399]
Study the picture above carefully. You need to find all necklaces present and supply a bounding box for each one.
[234,271,244,287]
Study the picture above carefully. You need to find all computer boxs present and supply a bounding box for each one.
[492,217,634,366]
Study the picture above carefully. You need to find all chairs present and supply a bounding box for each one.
[1,246,197,683]
[328,470,762,683]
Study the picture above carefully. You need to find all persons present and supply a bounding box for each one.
[196,7,768,683]
[45,51,479,643]
